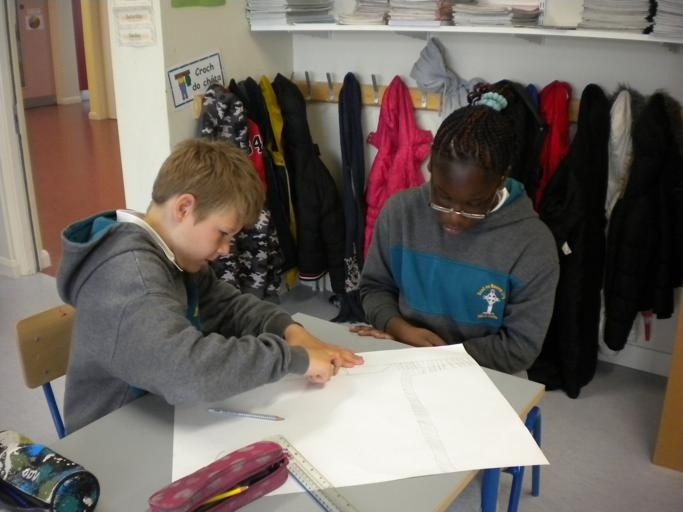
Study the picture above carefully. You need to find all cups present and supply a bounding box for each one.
[426,186,499,221]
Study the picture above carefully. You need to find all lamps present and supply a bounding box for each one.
[15,305,88,439]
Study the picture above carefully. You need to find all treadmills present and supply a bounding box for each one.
[274,436,364,512]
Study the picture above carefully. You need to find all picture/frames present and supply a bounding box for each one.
[0,308,550,512]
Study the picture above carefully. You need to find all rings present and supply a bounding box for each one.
[201,486,249,506]
[208,408,285,421]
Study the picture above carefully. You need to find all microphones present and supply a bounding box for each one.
[480,403,545,511]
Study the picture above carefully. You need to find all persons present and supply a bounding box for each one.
[55,136,366,438]
[348,78,561,381]
